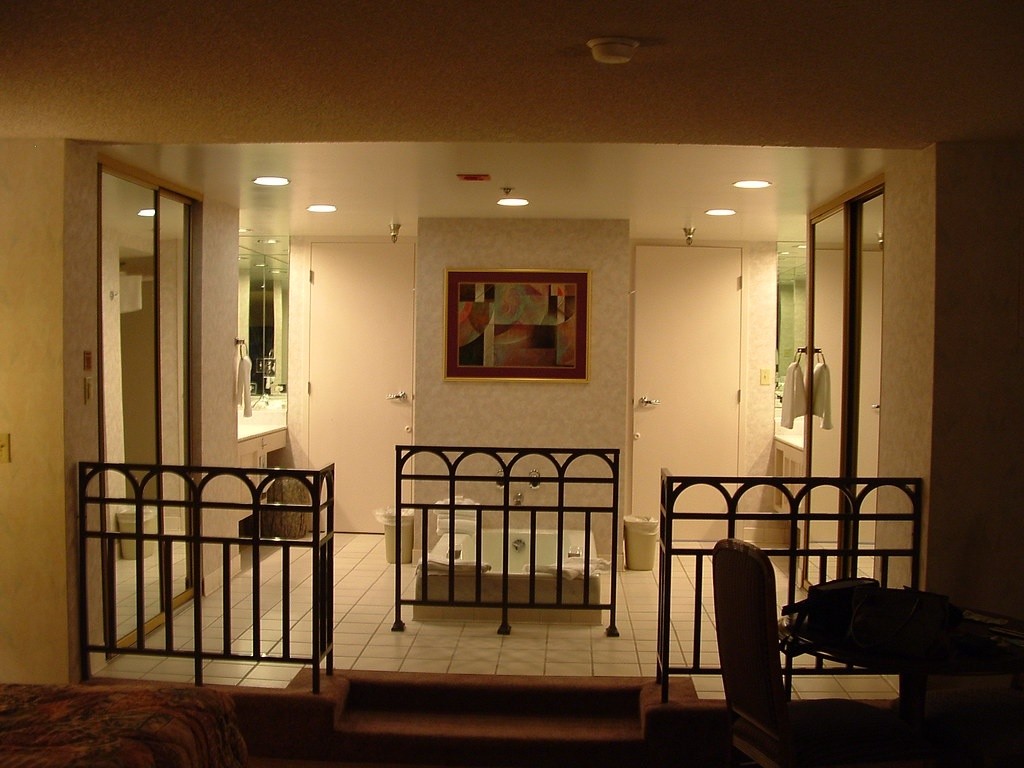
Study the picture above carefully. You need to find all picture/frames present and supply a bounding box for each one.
[442,267,593,384]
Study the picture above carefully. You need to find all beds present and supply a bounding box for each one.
[0,682,249,768]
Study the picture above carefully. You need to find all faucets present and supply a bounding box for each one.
[513,493,524,505]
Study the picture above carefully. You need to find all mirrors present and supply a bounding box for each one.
[847,192,883,580]
[99,169,163,642]
[159,194,191,601]
[774,240,807,434]
[801,201,846,592]
[238,245,290,397]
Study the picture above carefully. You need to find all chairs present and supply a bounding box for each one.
[712,538,921,768]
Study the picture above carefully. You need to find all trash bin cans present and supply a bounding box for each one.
[625,515,661,571]
[374,505,413,565]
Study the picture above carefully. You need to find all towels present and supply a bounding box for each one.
[434,496,481,535]
[780,362,805,429]
[416,553,492,576]
[812,364,833,430]
[234,356,253,417]
[523,557,610,579]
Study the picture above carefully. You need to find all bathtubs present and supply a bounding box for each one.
[414,527,603,625]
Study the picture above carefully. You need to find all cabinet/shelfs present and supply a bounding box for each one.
[772,434,805,531]
[237,429,287,522]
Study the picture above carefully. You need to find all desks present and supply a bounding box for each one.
[778,609,1024,768]
